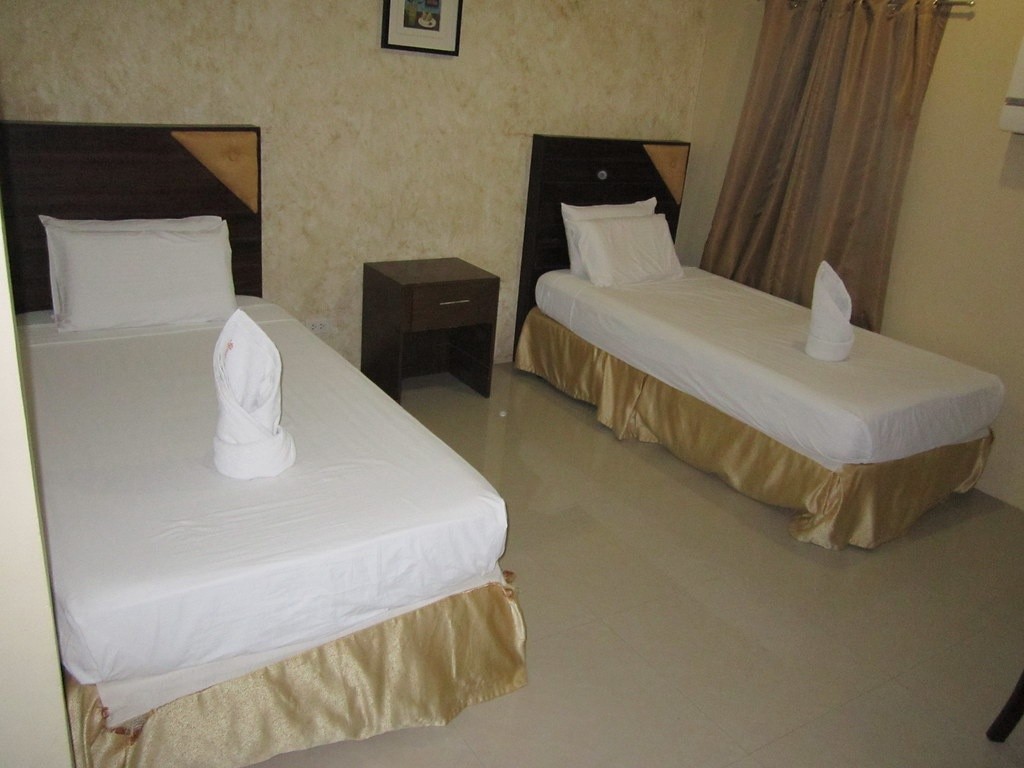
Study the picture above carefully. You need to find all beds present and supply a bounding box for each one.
[513,133,1006,550]
[0,119,528,768]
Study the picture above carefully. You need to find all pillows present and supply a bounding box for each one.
[574,213,685,287]
[562,197,657,278]
[38,215,239,333]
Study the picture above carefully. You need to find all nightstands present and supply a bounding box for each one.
[360,258,500,405]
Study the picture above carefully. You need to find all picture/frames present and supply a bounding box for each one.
[381,0,463,56]
